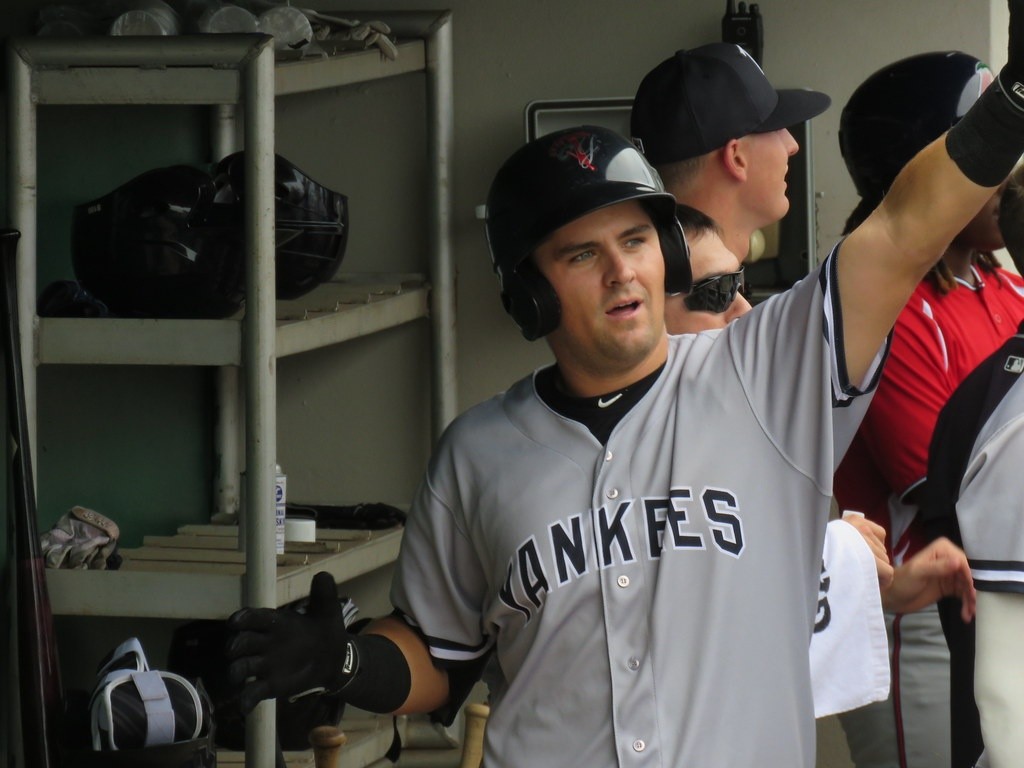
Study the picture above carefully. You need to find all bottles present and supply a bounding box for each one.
[275,463,287,555]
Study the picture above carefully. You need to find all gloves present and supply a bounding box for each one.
[311,10,398,60]
[351,502,406,530]
[997,0,1024,117]
[214,571,363,711]
[39,506,122,570]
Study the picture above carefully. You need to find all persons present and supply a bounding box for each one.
[629,41,833,263]
[666,204,976,768]
[226,0,1024,768]
[840,51,1024,768]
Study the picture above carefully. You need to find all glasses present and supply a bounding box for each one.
[683,268,753,315]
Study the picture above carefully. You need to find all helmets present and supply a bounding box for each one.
[70,165,237,318]
[484,126,693,340]
[203,148,348,303]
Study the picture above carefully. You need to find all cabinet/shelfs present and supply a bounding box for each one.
[0,6,460,768]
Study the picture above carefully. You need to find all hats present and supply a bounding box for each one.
[630,42,831,168]
[838,51,995,237]
